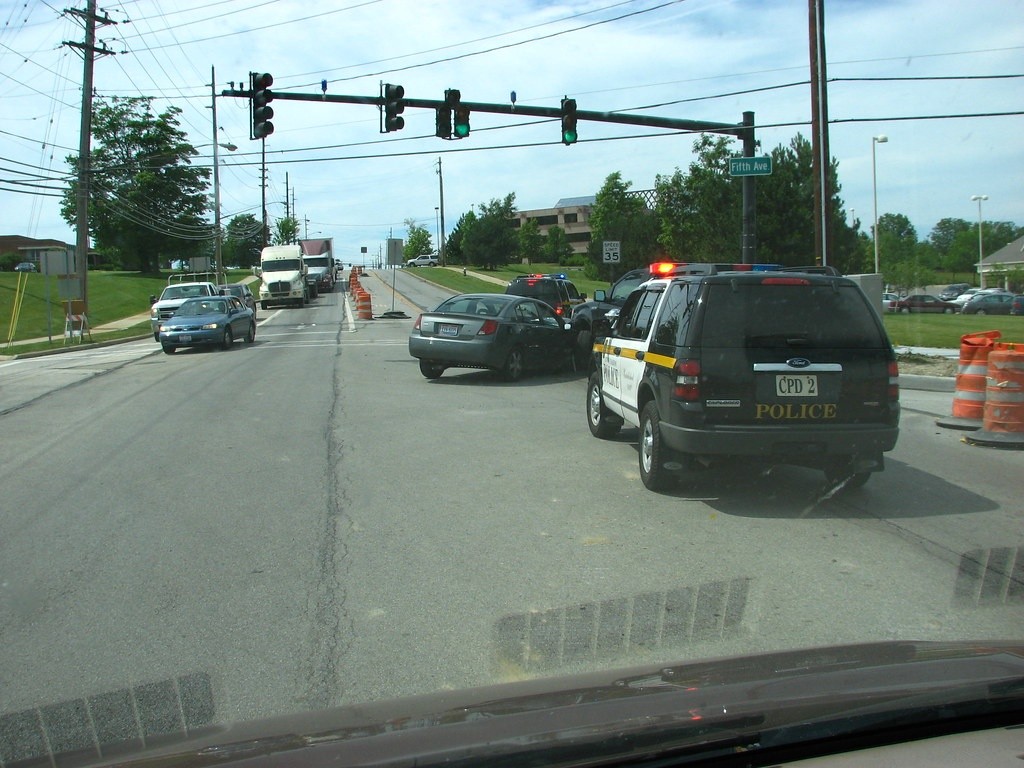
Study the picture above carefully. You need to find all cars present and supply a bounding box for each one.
[882,292,903,313]
[407,292,578,384]
[946,287,1024,316]
[888,294,962,315]
[938,283,971,301]
[160,295,257,355]
[15,262,38,273]
[334,259,344,274]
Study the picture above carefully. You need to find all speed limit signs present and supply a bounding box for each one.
[602,241,621,264]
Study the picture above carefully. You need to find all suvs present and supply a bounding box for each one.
[504,274,589,319]
[585,263,901,492]
[149,270,229,343]
[216,283,257,321]
[570,268,651,369]
[406,254,440,267]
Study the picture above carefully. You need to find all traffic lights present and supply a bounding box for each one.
[436,101,452,138]
[384,83,405,132]
[252,72,275,139]
[455,104,471,138]
[560,98,578,144]
[445,88,460,106]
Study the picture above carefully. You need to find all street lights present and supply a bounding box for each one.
[304,230,323,240]
[971,195,989,289]
[75,142,238,299]
[871,135,889,275]
[293,218,310,245]
[850,208,856,228]
[434,207,440,265]
[213,200,290,285]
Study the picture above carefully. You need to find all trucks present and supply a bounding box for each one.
[296,236,338,293]
[254,245,310,310]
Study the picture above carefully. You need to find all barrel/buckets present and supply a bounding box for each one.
[62,300,84,329]
[951,330,1024,432]
[348,265,372,319]
